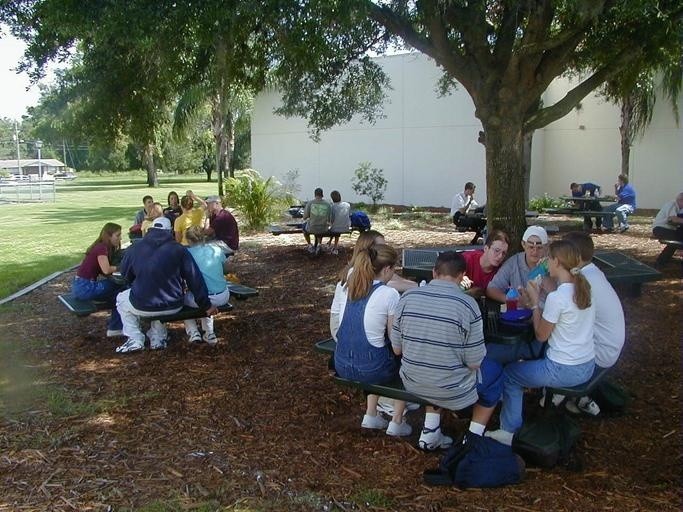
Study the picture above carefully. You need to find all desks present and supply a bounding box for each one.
[560,194,614,203]
[469,294,503,340]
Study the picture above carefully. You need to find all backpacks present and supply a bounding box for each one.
[351,211,370,233]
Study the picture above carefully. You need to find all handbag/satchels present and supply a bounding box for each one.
[129,224,142,233]
[596,380,626,408]
[512,419,562,467]
[423,435,527,490]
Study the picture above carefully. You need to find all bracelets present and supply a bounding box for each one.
[529,305,538,311]
[417,280,420,287]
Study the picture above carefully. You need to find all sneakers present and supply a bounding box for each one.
[106,330,123,337]
[376,399,409,417]
[360,414,388,429]
[418,425,453,451]
[406,401,421,411]
[149,337,168,350]
[462,435,468,445]
[202,333,218,344]
[605,227,614,232]
[620,226,629,233]
[188,331,202,343]
[386,418,412,436]
[566,397,581,414]
[577,395,600,416]
[484,429,514,447]
[539,393,565,408]
[115,338,143,353]
[331,247,339,255]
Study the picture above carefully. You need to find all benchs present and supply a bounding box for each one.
[401,244,485,279]
[542,207,575,215]
[566,209,617,218]
[314,337,336,353]
[268,222,282,235]
[453,225,479,243]
[333,376,438,405]
[286,220,304,229]
[595,248,659,285]
[221,281,257,299]
[159,302,233,323]
[57,290,96,315]
[314,229,351,238]
[658,237,682,250]
[548,362,609,397]
[545,226,558,234]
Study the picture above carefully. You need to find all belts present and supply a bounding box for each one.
[208,292,222,295]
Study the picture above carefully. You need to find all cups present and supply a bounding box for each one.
[505,288,519,311]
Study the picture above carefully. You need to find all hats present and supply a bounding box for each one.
[205,195,221,203]
[152,217,172,230]
[522,225,548,245]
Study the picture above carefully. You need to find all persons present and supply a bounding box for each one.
[330,231,420,418]
[653,192,682,270]
[162,192,182,233]
[173,190,207,246]
[487,225,548,310]
[203,195,238,255]
[450,182,487,245]
[483,239,595,447]
[333,244,412,438]
[539,232,626,416]
[140,202,171,237]
[135,195,152,225]
[302,188,330,256]
[183,225,230,344]
[570,182,600,230]
[114,217,217,353]
[601,174,635,233]
[329,190,350,255]
[389,250,504,451]
[71,223,122,336]
[450,230,511,290]
